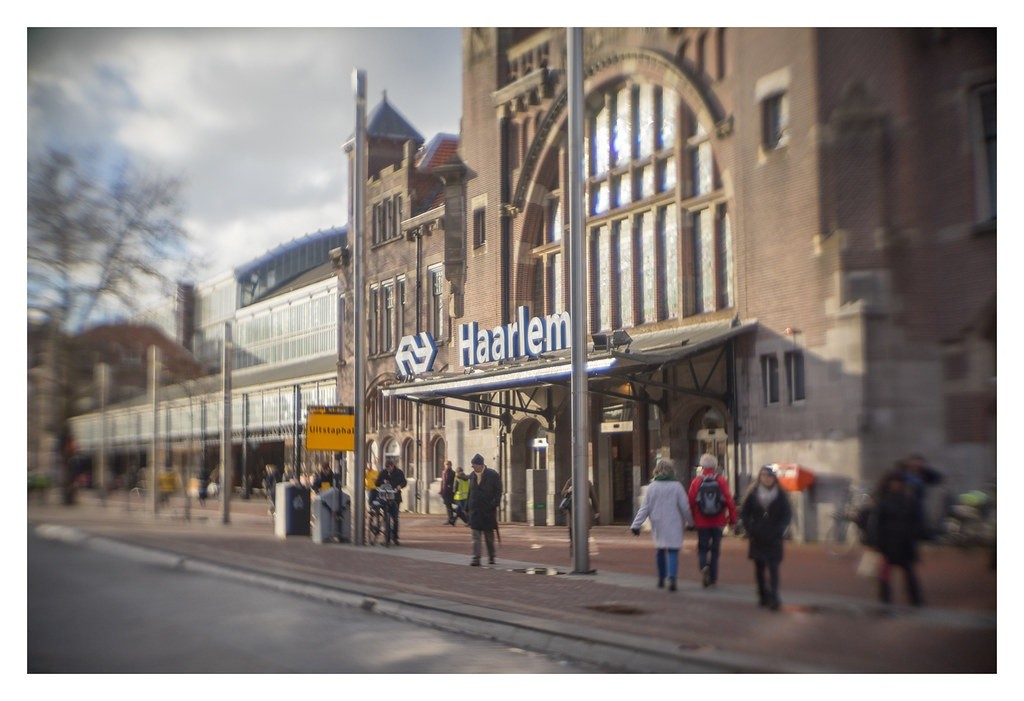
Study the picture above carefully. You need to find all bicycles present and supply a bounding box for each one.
[367,488,396,549]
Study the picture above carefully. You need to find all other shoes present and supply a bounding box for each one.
[658,579,664,585]
[671,580,677,590]
[701,565,712,586]
[771,598,779,609]
[711,577,718,582]
[489,556,495,563]
[471,558,480,565]
[758,593,767,604]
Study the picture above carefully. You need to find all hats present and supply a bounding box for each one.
[470,454,484,465]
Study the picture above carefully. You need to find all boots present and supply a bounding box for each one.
[879,580,892,605]
[908,582,922,606]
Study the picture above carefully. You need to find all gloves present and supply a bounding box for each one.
[686,526,695,531]
[632,528,640,535]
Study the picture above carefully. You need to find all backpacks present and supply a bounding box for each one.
[696,472,726,516]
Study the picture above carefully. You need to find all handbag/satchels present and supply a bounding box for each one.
[857,509,869,528]
[380,484,396,500]
[560,495,572,511]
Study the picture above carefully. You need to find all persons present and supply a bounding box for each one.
[872,457,943,606]
[740,465,792,611]
[365,459,407,545]
[631,458,694,591]
[311,461,334,494]
[562,476,600,550]
[439,460,457,525]
[448,467,470,526]
[687,454,738,588]
[262,464,293,517]
[464,454,503,566]
